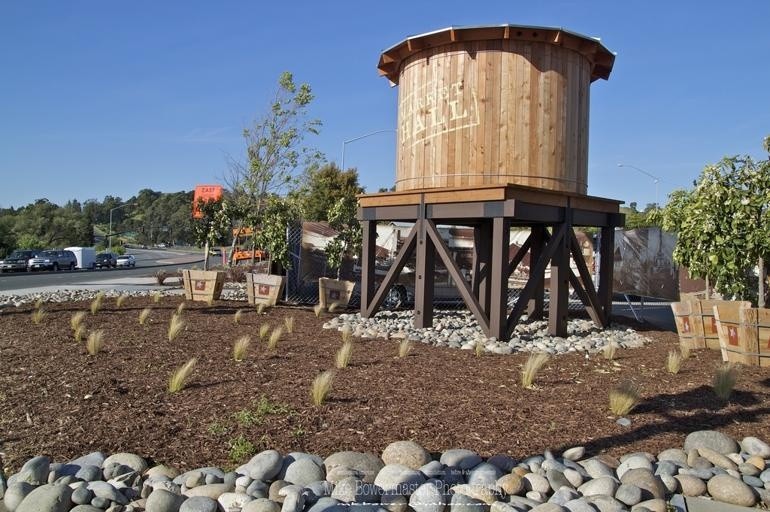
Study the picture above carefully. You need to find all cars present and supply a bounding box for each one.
[94,253,116,269]
[117,255,136,267]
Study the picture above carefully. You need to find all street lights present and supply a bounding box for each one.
[617,163,659,209]
[110,202,138,253]
[341,127,398,173]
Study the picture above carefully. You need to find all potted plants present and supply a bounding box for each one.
[639,136,770,372]
[181,197,380,313]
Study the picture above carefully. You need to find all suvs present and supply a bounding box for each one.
[28,250,77,272]
[0,250,40,272]
